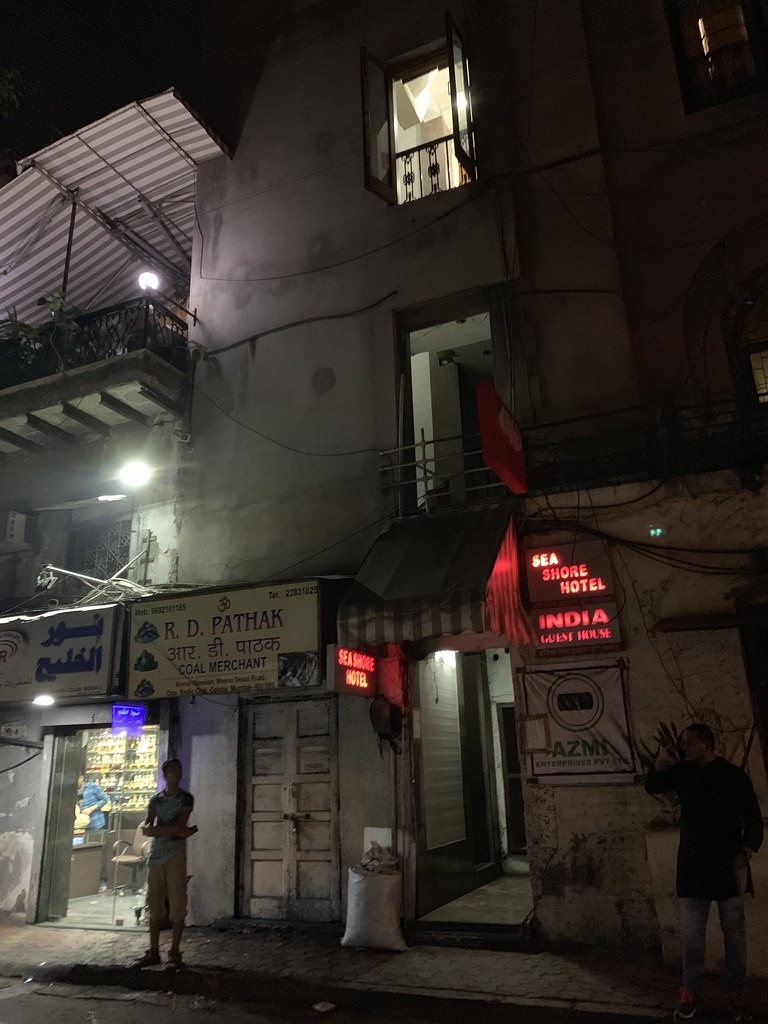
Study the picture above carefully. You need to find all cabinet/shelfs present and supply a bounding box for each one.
[86,728,158,813]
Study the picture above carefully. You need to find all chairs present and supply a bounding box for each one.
[108,821,157,897]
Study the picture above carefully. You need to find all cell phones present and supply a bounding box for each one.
[189,825,198,834]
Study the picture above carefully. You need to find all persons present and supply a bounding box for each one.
[645,723,763,1024]
[135,759,195,976]
[77,771,108,894]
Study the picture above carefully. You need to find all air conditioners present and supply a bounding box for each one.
[0,510,37,554]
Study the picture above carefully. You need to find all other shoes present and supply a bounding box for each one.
[98,886,107,893]
[130,947,161,967]
[166,949,184,969]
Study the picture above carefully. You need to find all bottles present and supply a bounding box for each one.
[87,735,157,808]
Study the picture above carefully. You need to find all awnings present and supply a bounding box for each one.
[338,517,537,656]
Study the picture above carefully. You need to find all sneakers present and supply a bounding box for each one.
[671,987,699,1018]
[728,989,752,1022]
